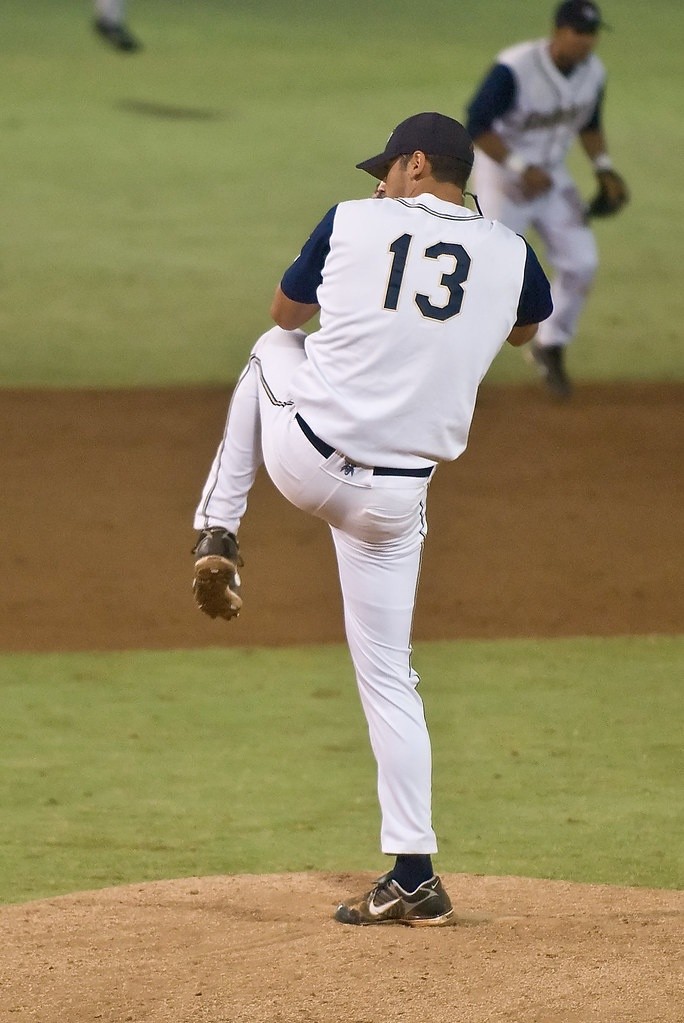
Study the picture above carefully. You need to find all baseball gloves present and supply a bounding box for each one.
[586,168,627,217]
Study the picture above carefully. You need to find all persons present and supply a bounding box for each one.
[465,0,631,391]
[191,113,554,928]
[94,0,142,53]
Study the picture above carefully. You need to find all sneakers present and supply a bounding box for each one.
[336,869,455,927]
[192,526,244,621]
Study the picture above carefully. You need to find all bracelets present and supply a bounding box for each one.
[592,155,612,174]
[502,155,529,188]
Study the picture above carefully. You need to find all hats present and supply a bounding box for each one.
[556,0,608,32]
[355,112,475,181]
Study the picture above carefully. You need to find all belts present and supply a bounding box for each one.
[296,413,434,477]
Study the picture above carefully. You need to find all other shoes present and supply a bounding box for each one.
[534,345,570,391]
